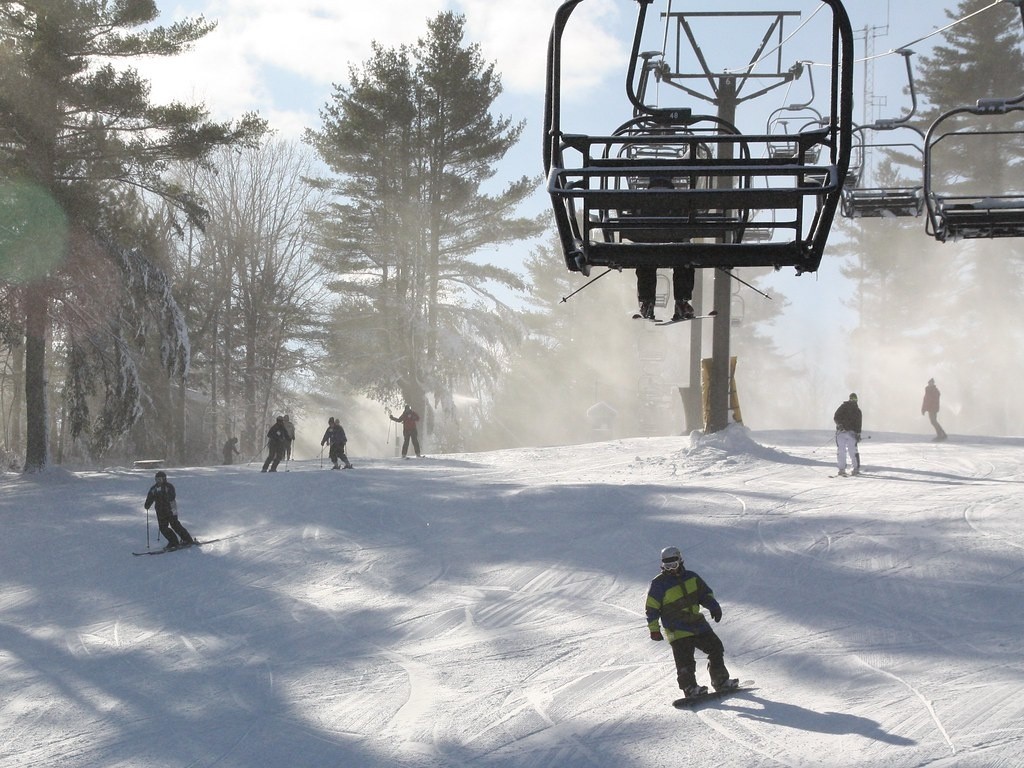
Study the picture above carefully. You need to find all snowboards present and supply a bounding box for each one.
[672,678,757,707]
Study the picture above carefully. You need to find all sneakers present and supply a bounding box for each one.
[715,678,739,691]
[684,684,708,698]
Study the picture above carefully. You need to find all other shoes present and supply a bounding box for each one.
[345,464,351,467]
[181,537,192,546]
[333,465,338,469]
[166,540,179,548]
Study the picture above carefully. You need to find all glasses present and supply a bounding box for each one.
[662,562,679,570]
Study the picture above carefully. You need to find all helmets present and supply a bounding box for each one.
[661,547,684,564]
[849,393,857,400]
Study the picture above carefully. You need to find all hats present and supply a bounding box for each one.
[155,472,167,481]
[928,379,935,384]
[405,405,411,410]
[284,415,289,420]
[276,416,284,421]
[329,417,334,423]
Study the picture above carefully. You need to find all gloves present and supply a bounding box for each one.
[711,605,722,623]
[650,631,663,641]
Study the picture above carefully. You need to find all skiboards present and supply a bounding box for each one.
[132,539,220,555]
[655,310,718,325]
[632,314,661,323]
[828,473,860,477]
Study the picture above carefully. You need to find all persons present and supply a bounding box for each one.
[630,177,695,322]
[833,393,863,476]
[222,437,239,464]
[261,415,295,471]
[921,378,948,442]
[390,405,421,457]
[144,471,193,550]
[645,546,739,695]
[320,417,350,469]
